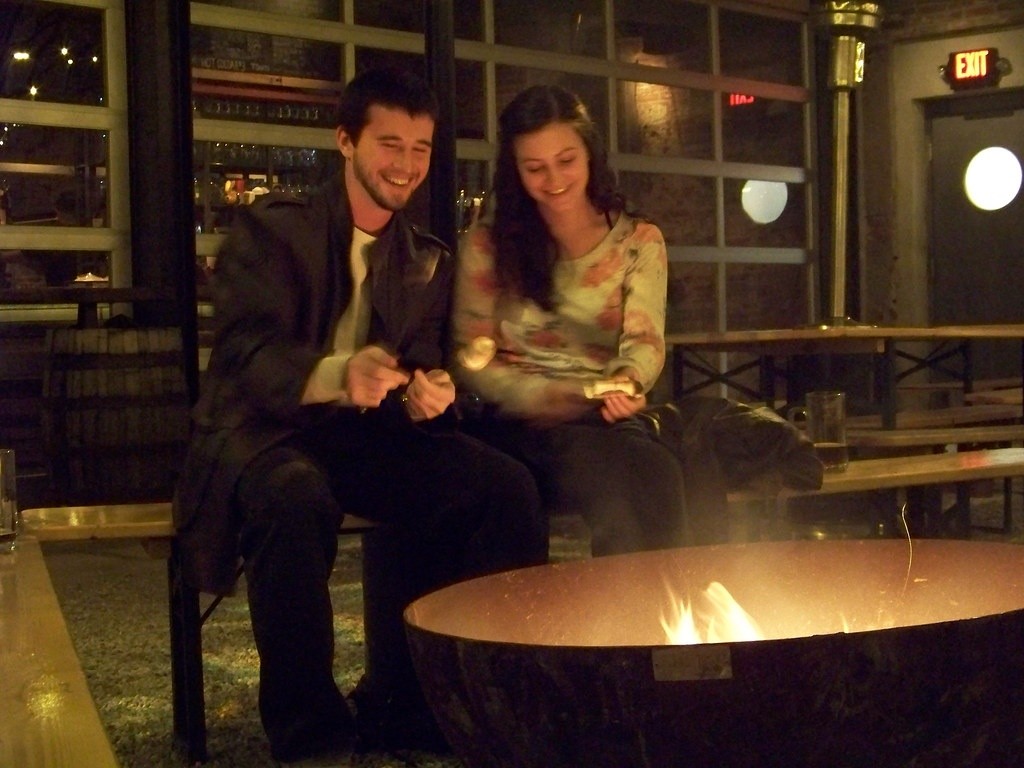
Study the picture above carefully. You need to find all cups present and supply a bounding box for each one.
[0,449,21,551]
[194,99,320,206]
[787,391,849,474]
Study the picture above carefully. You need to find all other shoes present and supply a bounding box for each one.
[360,681,454,754]
[258,688,356,761]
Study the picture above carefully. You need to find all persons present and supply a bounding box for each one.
[173,72,540,762]
[455,87,726,554]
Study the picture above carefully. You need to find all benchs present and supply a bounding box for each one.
[721,388,1024,508]
[19,504,367,759]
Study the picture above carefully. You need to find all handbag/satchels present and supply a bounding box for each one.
[611,395,825,491]
